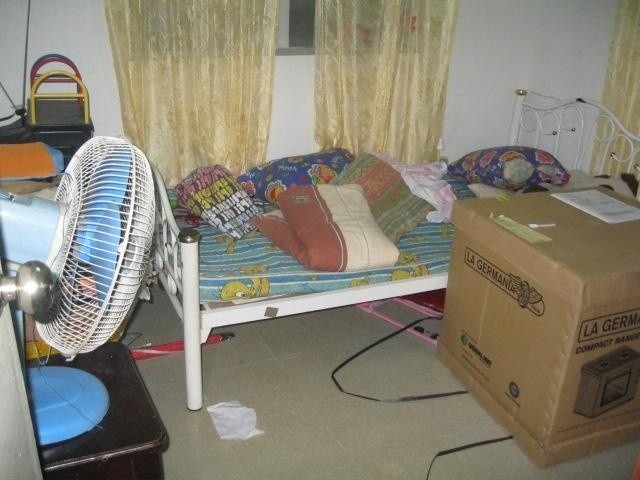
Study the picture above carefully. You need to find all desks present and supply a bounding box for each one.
[26,340,169,480]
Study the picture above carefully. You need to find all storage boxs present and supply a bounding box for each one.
[435,186,640,469]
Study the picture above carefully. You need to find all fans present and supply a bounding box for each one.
[0,135,156,447]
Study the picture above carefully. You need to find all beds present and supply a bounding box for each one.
[150,88,639,411]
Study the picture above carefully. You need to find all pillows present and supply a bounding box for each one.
[237,148,354,206]
[447,146,571,190]
